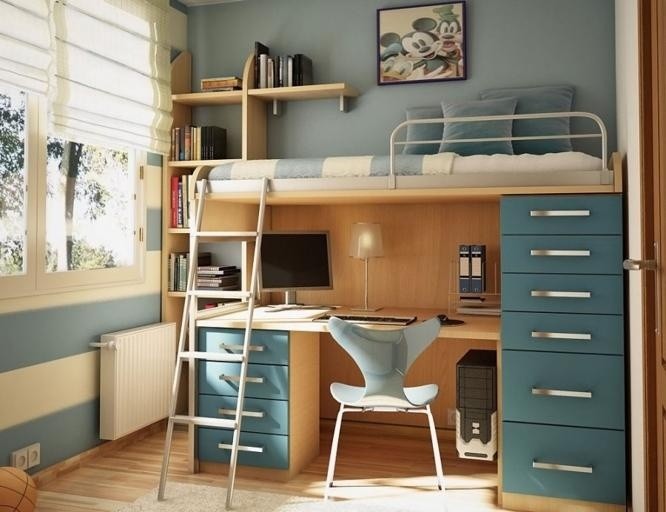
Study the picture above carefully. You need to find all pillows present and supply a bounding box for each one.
[481,86,574,152]
[401,106,446,153]
[438,96,518,155]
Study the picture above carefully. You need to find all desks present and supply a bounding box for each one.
[189,305,502,506]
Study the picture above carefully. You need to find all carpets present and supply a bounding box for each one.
[114,480,445,511]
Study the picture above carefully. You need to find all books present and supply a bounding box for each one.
[169,251,240,292]
[203,302,232,309]
[200,76,243,91]
[172,125,226,160]
[171,174,192,229]
[255,39,314,89]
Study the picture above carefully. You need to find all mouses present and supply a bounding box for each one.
[437,314,449,322]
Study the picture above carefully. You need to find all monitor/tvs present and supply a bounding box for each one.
[256,230,334,313]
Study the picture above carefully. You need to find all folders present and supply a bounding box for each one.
[458,245,471,293]
[472,245,487,293]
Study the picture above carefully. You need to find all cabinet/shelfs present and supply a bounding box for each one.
[497,194,634,512]
[157,50,268,413]
[197,328,323,483]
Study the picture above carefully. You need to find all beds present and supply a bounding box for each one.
[190,153,628,204]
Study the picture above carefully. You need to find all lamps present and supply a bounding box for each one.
[349,221,385,311]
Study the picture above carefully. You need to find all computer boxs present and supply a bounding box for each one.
[455,348,498,462]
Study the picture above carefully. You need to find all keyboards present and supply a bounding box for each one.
[312,314,417,326]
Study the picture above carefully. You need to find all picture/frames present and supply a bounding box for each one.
[376,0,467,85]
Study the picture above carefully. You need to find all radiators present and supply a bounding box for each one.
[100,321,177,440]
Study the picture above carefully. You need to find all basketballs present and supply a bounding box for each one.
[0,465,36,512]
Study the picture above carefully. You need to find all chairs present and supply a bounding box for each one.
[324,317,446,490]
